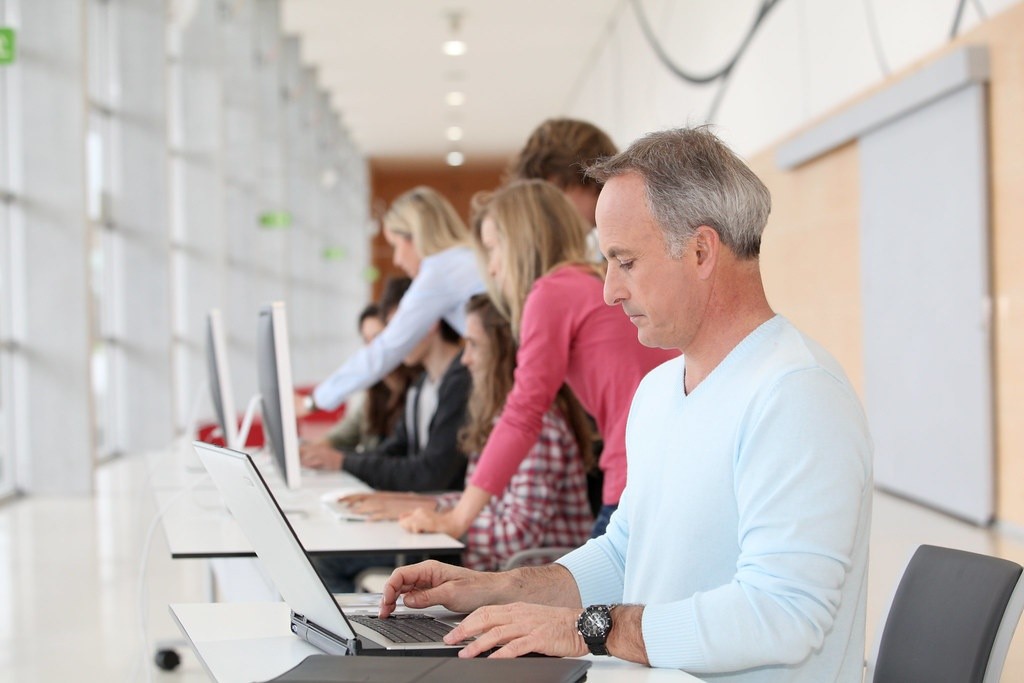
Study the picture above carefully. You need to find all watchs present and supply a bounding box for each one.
[575,603,615,655]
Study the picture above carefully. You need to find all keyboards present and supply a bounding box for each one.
[318,490,370,523]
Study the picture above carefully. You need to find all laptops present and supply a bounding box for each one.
[193,440,562,660]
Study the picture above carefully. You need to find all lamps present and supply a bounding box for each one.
[437,14,470,58]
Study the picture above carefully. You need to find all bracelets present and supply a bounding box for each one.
[304,395,317,412]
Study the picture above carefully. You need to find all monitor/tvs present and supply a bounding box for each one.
[205,309,239,448]
[229,302,304,513]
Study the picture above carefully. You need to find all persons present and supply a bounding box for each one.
[510,120,619,261]
[378,128,873,683]
[293,186,497,421]
[301,275,474,590]
[402,179,682,542]
[317,304,412,455]
[339,290,593,570]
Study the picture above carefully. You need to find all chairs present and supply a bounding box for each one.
[867,544,1024,683]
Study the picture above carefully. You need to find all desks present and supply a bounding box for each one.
[168,601,707,683]
[150,464,467,558]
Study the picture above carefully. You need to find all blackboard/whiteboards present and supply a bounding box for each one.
[856,77,1002,529]
